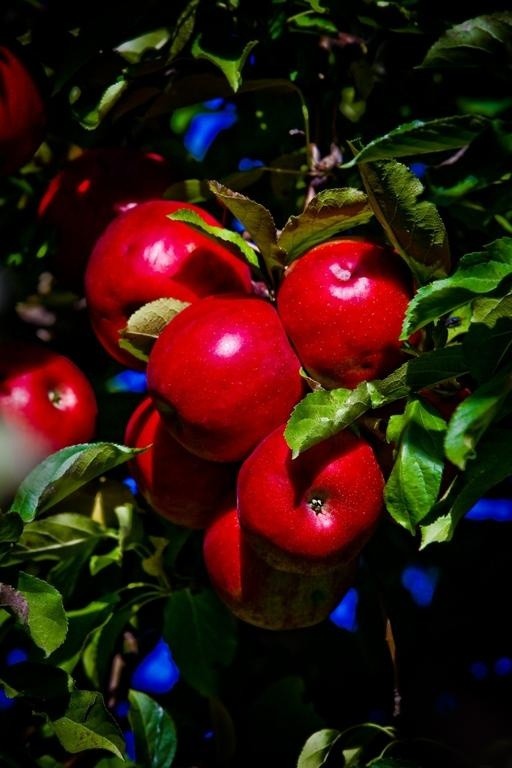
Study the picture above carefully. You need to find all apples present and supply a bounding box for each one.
[203,493,353,631]
[0,46,47,175]
[125,399,226,529]
[0,346,98,510]
[37,147,165,266]
[277,236,426,390]
[85,195,256,373]
[235,422,386,560]
[145,290,305,465]
